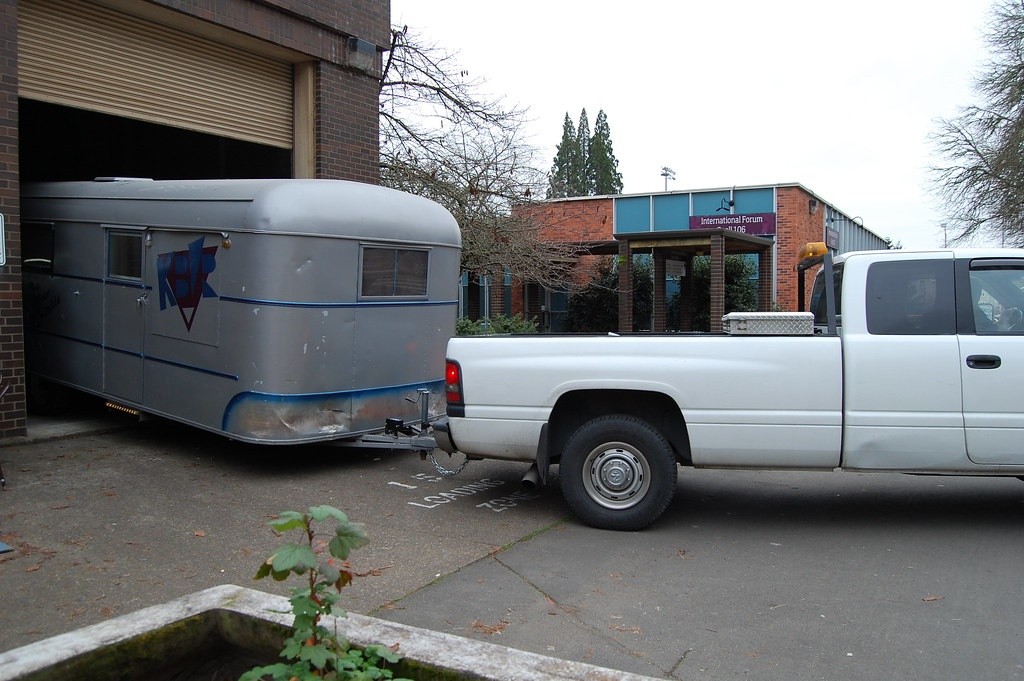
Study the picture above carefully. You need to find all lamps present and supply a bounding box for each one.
[831,216,863,229]
[345,37,376,72]
[716,198,735,211]
[810,199,819,214]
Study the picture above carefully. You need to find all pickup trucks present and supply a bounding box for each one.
[428,242,1024,530]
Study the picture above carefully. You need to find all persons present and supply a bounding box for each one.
[970,277,1013,332]
